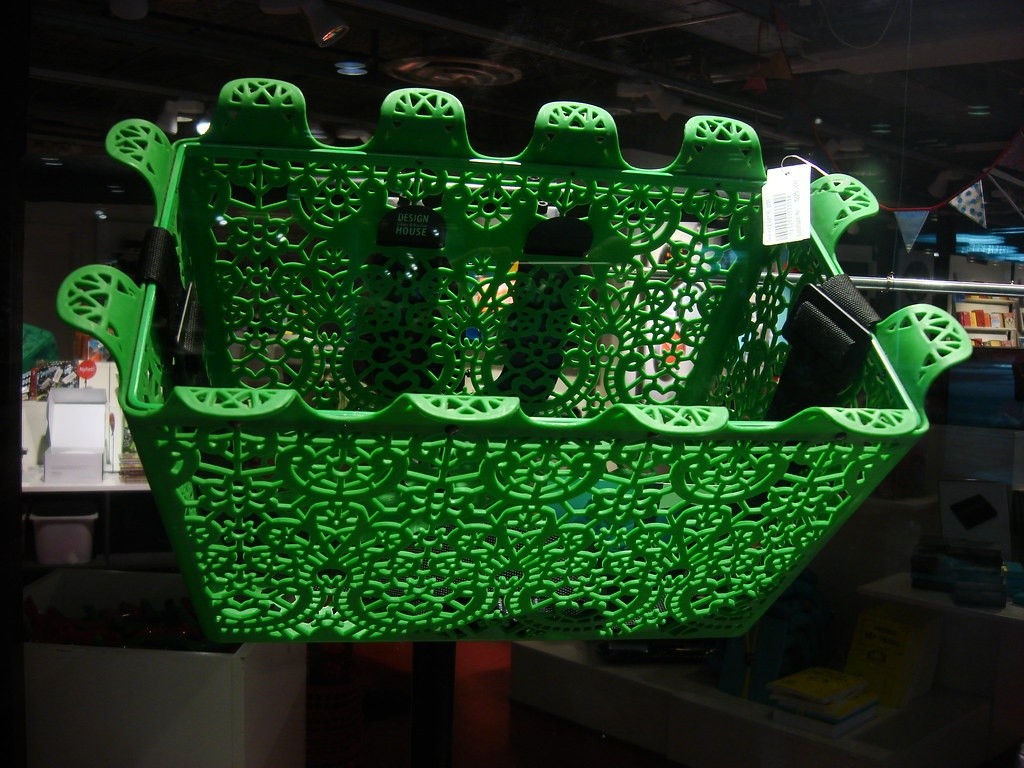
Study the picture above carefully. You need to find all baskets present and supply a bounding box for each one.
[53,71,973,650]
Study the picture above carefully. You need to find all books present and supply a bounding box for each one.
[765,605,944,739]
[909,533,1024,607]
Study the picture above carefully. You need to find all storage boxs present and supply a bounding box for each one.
[22,569,307,768]
[56,78,973,648]
[28,503,99,567]
